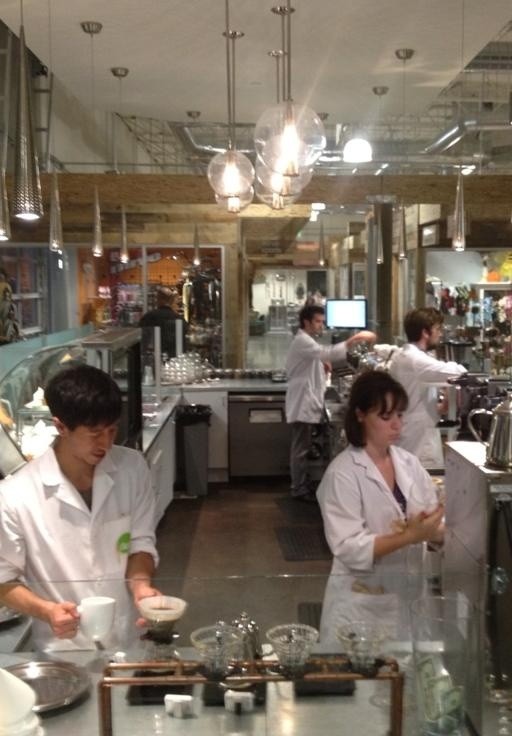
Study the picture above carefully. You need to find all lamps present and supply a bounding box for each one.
[255,1,326,211]
[1,24,13,243]
[80,20,104,258]
[184,109,203,266]
[12,0,45,223]
[47,0,66,254]
[206,0,255,215]
[340,46,465,256]
[110,67,130,265]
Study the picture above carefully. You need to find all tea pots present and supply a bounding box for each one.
[467,392,512,466]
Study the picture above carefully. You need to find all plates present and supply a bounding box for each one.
[2,660,90,717]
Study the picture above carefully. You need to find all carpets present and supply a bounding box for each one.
[299,601,323,630]
[272,494,323,523]
[275,524,333,562]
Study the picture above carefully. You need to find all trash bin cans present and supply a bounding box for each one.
[176,405,213,497]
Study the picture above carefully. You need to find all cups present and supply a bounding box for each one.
[335,622,391,673]
[266,624,320,673]
[344,342,392,376]
[190,626,243,677]
[409,596,478,735]
[74,596,116,644]
[215,369,271,378]
[0,668,44,736]
[136,594,190,642]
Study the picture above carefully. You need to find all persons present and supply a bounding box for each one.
[284,306,377,502]
[1,364,161,653]
[315,372,445,646]
[387,307,468,470]
[139,287,188,358]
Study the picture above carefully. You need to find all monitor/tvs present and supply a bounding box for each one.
[325,299,369,329]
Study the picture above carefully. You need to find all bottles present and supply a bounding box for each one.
[230,612,260,661]
[159,352,214,385]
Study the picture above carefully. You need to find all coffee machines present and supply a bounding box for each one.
[440,441,511,735]
